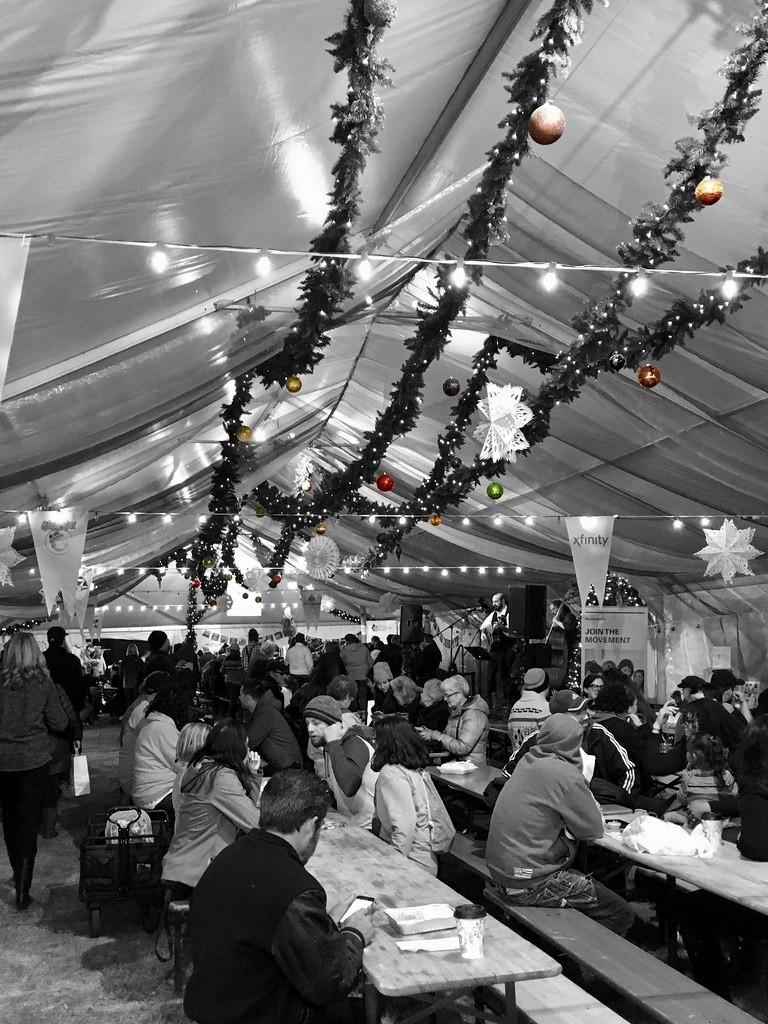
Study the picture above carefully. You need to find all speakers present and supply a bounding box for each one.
[400,604,423,644]
[508,585,547,639]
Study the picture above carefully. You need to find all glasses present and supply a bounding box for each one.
[445,692,459,697]
[590,685,605,690]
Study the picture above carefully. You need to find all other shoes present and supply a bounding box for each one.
[16,899,24,909]
[22,896,32,908]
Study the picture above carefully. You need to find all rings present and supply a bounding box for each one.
[691,819,695,822]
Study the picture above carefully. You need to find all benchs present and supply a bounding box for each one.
[483,881,768,1023]
[430,833,495,879]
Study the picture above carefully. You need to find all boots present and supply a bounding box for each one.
[43,809,59,839]
[39,807,47,835]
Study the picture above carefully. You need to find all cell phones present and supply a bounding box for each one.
[606,819,629,828]
[338,895,375,925]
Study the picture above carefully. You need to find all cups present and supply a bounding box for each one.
[700,812,724,847]
[453,904,487,959]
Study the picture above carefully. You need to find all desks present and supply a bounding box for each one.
[252,776,563,1024]
[575,812,768,968]
[423,761,502,836]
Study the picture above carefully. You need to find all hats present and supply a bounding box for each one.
[143,672,178,692]
[523,668,546,689]
[303,695,343,726]
[549,690,590,715]
[229,644,240,651]
[248,629,259,637]
[47,626,69,639]
[711,670,744,686]
[373,661,394,681]
[148,631,167,651]
[677,675,706,688]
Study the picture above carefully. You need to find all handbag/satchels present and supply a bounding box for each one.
[71,748,92,796]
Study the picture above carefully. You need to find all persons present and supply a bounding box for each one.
[585,659,644,700]
[582,674,738,829]
[42,682,81,841]
[183,768,381,1024]
[501,690,634,900]
[508,668,551,753]
[41,627,82,761]
[686,713,768,862]
[104,665,118,719]
[419,675,490,834]
[484,712,635,973]
[711,669,754,727]
[81,666,101,725]
[0,631,69,910]
[119,627,437,876]
[550,600,578,690]
[118,644,145,707]
[479,593,515,711]
